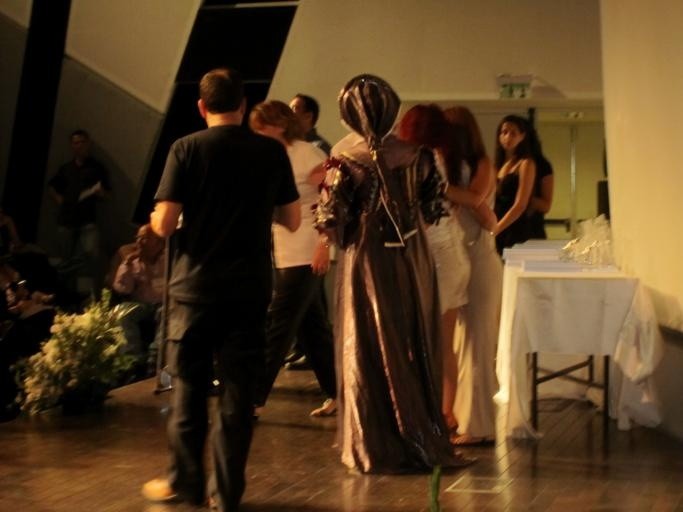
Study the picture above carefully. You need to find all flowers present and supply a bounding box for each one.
[15,288,143,414]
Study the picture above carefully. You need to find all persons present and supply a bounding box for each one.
[282,94,331,371]
[0,213,78,314]
[240,92,336,417]
[108,220,167,349]
[398,102,496,437]
[147,65,301,511]
[312,69,474,476]
[441,106,505,446]
[487,116,537,263]
[523,125,554,242]
[44,128,111,258]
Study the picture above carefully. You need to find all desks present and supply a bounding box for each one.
[496,240,651,437]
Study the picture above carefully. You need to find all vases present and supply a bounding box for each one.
[60,379,110,423]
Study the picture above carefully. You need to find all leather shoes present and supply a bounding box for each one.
[141,478,179,502]
